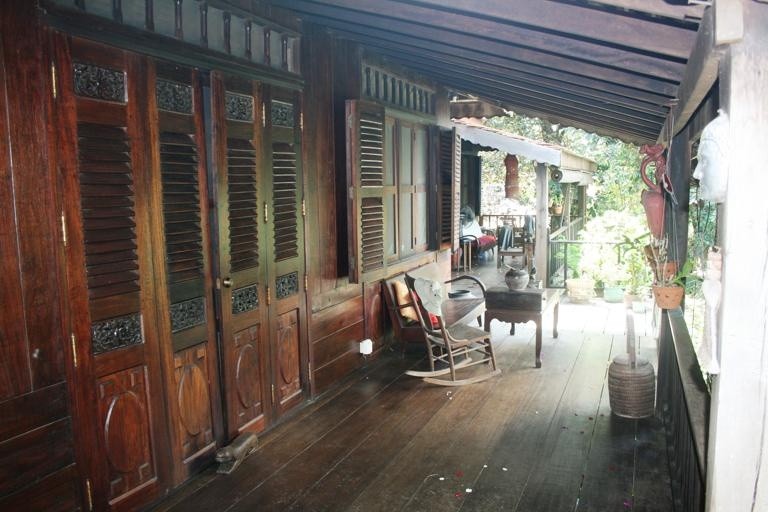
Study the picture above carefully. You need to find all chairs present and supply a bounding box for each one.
[382,269,503,388]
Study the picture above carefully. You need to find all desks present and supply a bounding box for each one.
[485,286,561,370]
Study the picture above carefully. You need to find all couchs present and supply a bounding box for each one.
[460,217,497,265]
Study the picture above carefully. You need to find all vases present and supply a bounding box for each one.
[644,243,685,310]
[565,279,625,303]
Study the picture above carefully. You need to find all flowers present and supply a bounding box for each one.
[598,260,629,287]
